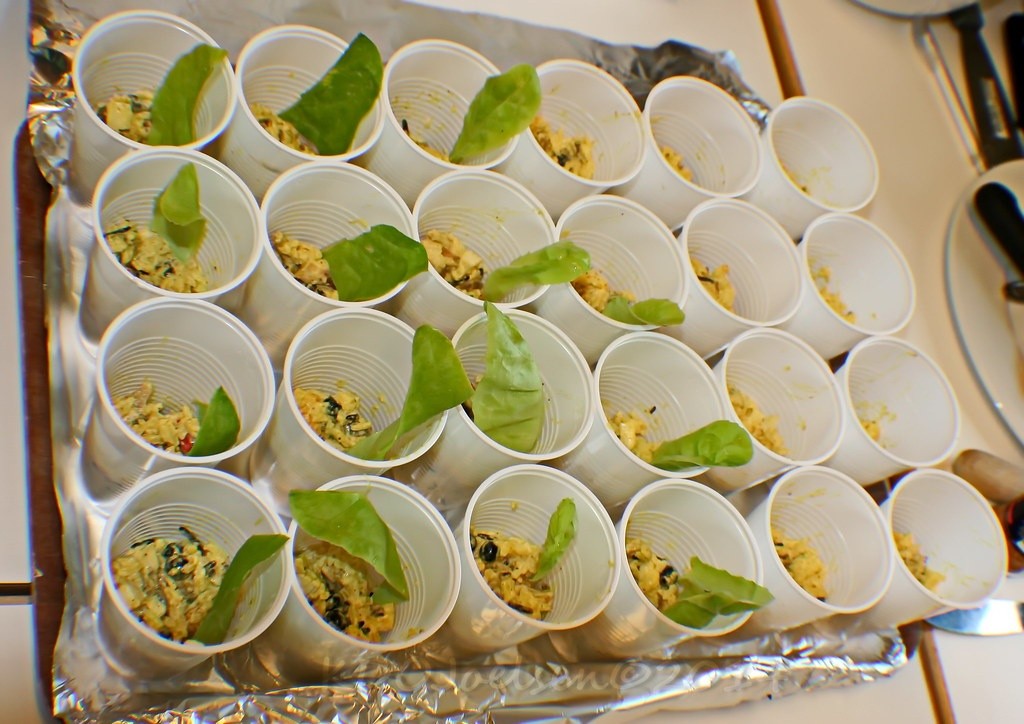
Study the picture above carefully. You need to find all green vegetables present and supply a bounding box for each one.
[147,32,779,646]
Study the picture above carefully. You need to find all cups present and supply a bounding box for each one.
[236,22,463,676]
[644,75,896,634]
[71,8,292,681]
[384,37,622,656]
[767,96,1008,626]
[536,58,764,659]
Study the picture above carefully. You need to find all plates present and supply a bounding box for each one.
[945,160,1024,446]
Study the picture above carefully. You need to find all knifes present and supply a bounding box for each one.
[919,599,1024,636]
